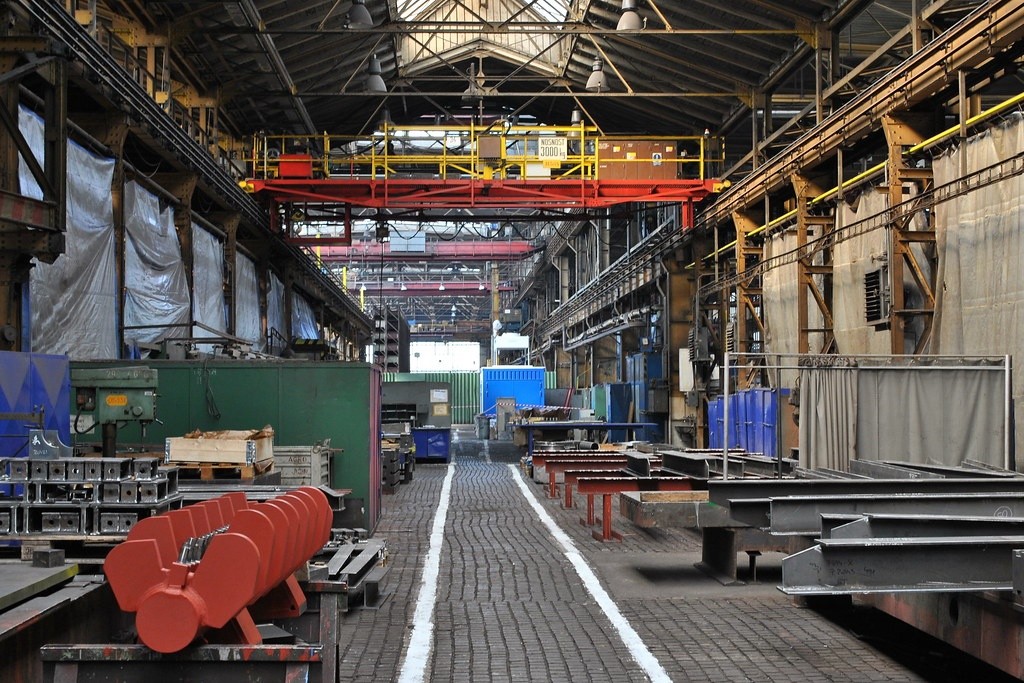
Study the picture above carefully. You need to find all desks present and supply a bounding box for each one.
[520,423,643,456]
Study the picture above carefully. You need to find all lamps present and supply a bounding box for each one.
[366,51,387,91]
[586,50,611,92]
[567,103,590,137]
[462,63,483,102]
[342,0,374,29]
[616,0,647,37]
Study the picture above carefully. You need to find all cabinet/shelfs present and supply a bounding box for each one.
[381,404,418,427]
[597,141,678,179]
[412,428,451,463]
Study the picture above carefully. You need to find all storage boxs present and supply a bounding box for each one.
[166,437,274,463]
